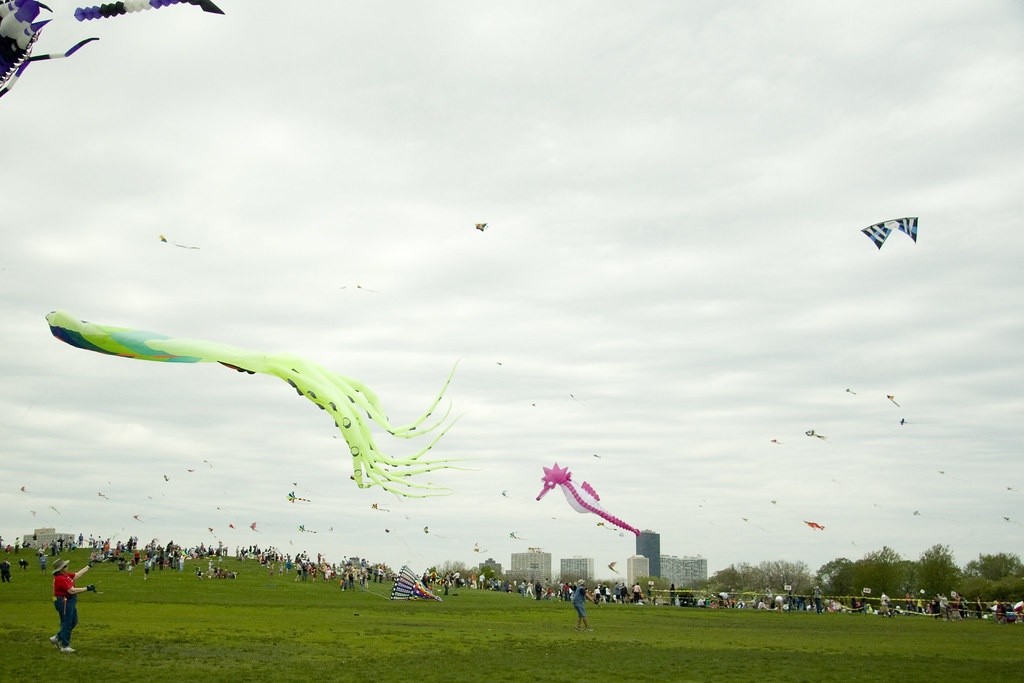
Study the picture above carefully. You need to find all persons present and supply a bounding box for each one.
[49,558,98,651]
[0,532,1024,626]
[573,579,599,632]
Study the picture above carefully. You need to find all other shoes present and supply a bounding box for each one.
[584,628,593,632]
[575,628,581,632]
[49,637,59,649]
[60,647,76,652]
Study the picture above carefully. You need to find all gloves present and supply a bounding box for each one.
[87,584,97,592]
[88,558,102,567]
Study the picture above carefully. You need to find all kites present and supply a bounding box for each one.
[537,462,641,536]
[390,565,443,602]
[46,310,471,497]
[742,388,1013,531]
[21,455,620,574]
[0,0,226,100]
[860,217,918,251]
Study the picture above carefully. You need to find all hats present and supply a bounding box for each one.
[52,558,70,574]
[578,579,585,585]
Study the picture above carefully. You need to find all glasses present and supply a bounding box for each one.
[64,565,67,568]
